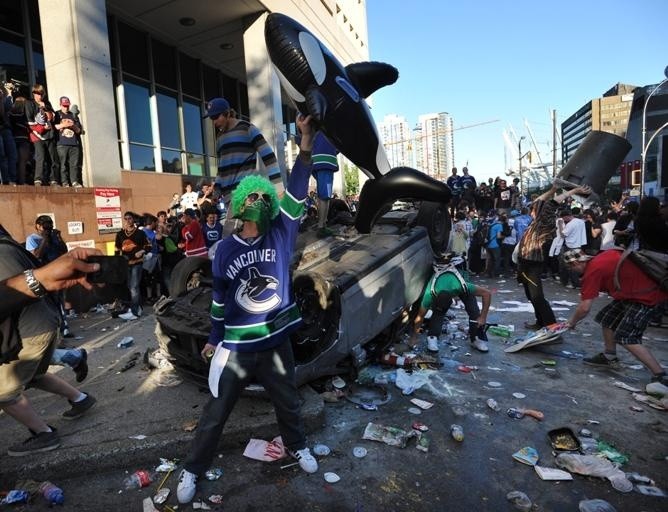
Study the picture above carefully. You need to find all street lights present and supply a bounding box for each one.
[516,134,528,205]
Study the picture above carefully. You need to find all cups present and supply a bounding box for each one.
[410,398,434,410]
[512,447,539,466]
[540,359,557,365]
[533,465,573,481]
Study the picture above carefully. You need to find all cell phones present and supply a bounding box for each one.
[86,255,128,284]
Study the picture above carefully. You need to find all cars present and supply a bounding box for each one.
[153,192,457,403]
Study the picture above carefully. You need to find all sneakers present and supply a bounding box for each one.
[288,447,318,473]
[8,180,82,187]
[426,336,439,352]
[8,428,60,456]
[526,322,540,330]
[472,337,488,352]
[176,468,198,504]
[583,353,618,367]
[317,227,331,239]
[645,373,668,395]
[63,392,97,419]
[73,349,88,382]
[119,305,143,320]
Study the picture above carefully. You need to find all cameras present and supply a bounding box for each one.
[2,78,28,91]
[46,230,59,237]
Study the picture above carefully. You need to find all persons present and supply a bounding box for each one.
[311,132,339,238]
[560,248,666,386]
[0,236,99,457]
[26,215,88,381]
[443,167,667,329]
[115,181,225,320]
[0,80,85,187]
[407,268,491,362]
[202,98,282,241]
[175,110,320,505]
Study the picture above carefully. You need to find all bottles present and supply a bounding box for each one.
[487,399,501,412]
[450,423,465,442]
[381,353,411,366]
[117,336,134,348]
[488,327,511,337]
[121,470,153,491]
[38,481,65,504]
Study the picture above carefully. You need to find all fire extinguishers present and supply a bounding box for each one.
[380,353,419,368]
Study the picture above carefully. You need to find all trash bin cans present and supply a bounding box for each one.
[554,131,632,201]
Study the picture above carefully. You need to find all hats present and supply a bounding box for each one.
[511,211,519,217]
[61,97,70,107]
[202,98,229,119]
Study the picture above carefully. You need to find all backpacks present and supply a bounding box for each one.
[478,224,492,246]
[615,249,667,295]
